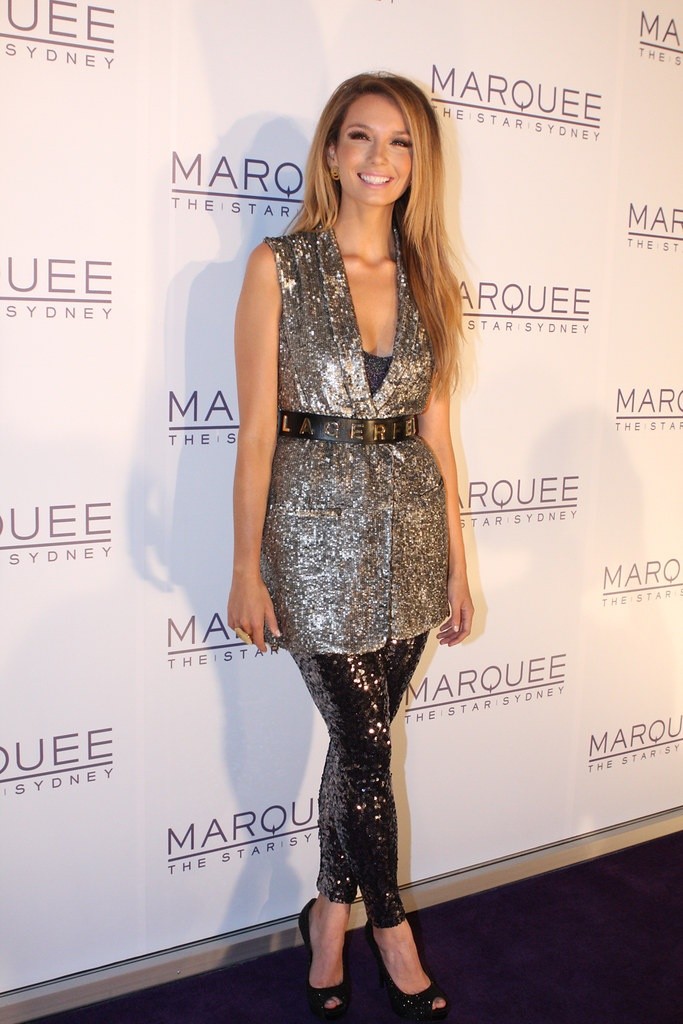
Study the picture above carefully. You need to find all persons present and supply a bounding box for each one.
[225,68,476,1022]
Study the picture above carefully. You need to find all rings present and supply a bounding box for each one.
[233,627,253,646]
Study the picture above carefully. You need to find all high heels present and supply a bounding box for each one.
[365,916,449,1022]
[298,898,349,1018]
